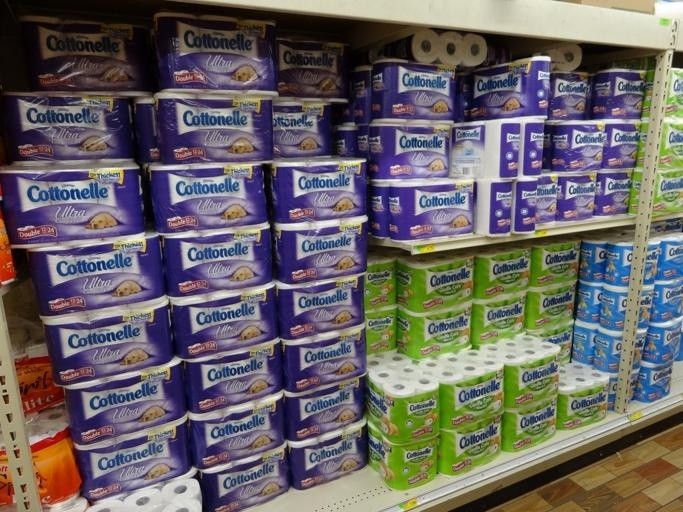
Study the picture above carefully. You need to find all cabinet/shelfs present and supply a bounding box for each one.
[0,0,683,512]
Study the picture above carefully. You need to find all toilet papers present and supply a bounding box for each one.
[0,0,683,511]
